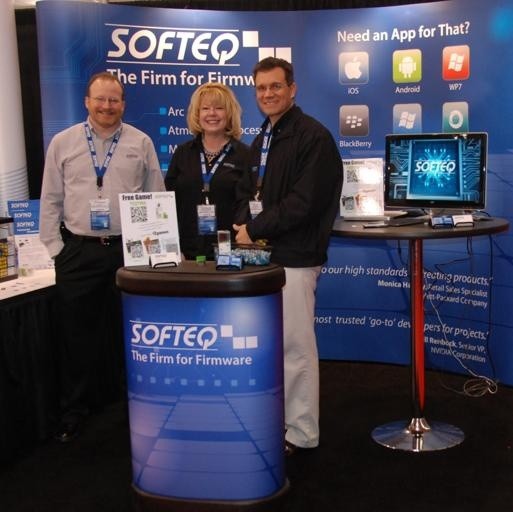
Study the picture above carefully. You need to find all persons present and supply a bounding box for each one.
[227,57,344,487]
[163,81,252,262]
[36,70,167,444]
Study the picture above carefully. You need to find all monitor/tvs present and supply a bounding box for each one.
[384,132,494,226]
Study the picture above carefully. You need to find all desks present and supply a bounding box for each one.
[326,210,511,454]
[0,219,60,447]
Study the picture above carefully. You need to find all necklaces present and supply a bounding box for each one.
[199,144,223,162]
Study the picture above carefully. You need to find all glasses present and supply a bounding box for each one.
[255,83,287,90]
[90,96,124,104]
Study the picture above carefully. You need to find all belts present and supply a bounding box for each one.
[63,231,122,246]
